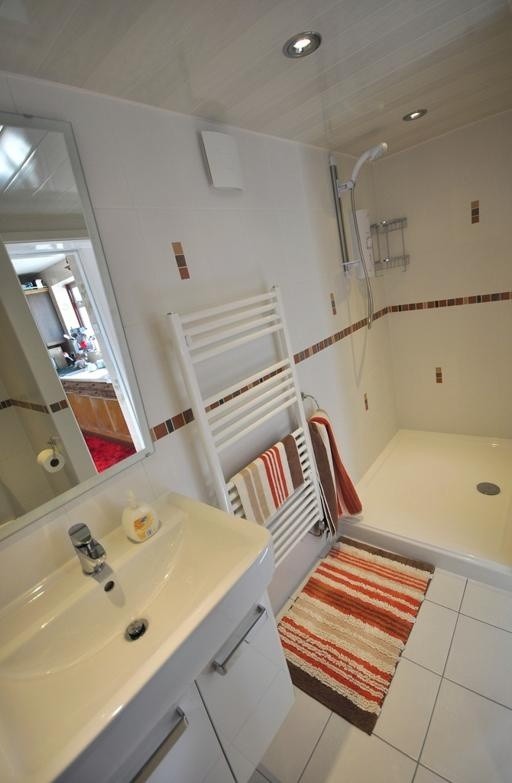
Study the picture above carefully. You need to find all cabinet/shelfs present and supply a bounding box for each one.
[62,382,134,444]
[107,589,294,783]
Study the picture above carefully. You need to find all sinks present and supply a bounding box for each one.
[0,491,277,783]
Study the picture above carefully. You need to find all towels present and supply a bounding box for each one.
[303,395,363,535]
[238,431,304,525]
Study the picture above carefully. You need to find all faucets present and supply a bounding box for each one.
[69,523,106,573]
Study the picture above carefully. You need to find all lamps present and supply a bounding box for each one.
[201,129,245,191]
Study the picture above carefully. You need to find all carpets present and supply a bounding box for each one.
[83,432,136,472]
[275,533,437,737]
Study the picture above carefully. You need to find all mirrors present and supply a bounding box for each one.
[0,110,154,536]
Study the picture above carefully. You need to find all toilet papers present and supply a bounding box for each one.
[36,448,65,474]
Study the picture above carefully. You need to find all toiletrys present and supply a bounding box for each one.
[120,485,159,542]
